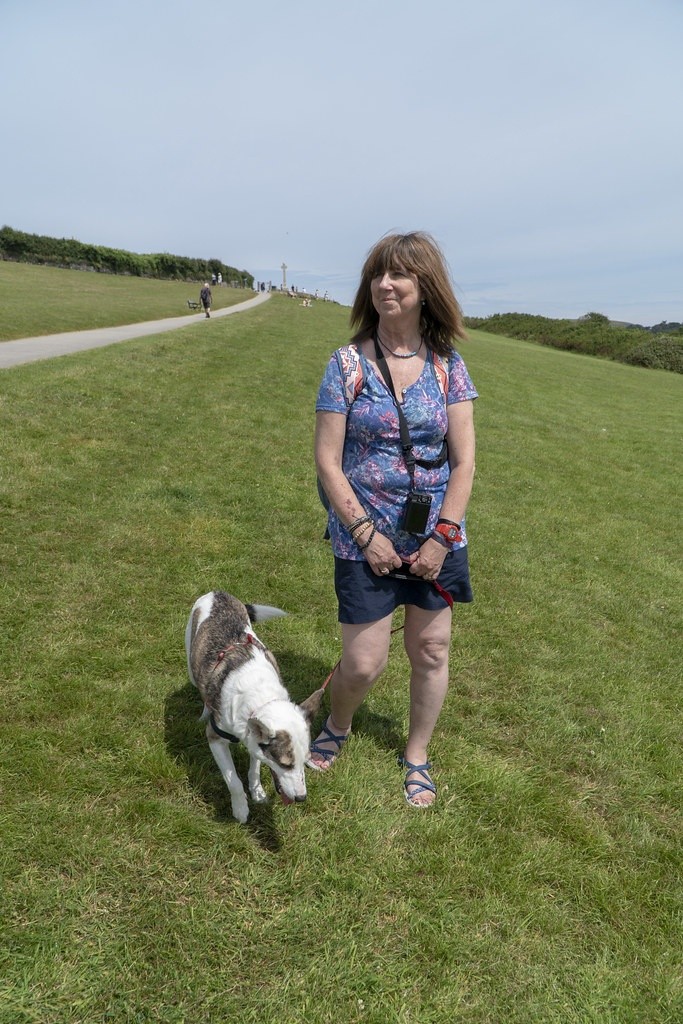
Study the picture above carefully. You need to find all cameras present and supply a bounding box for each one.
[402,491,432,534]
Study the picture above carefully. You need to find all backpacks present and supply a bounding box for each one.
[201,288,209,300]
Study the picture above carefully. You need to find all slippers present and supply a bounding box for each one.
[403,751,437,809]
[305,725,348,773]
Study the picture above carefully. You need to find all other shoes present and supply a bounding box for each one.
[205,313,210,318]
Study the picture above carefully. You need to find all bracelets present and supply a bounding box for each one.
[346,516,376,550]
[436,518,461,531]
[430,532,453,549]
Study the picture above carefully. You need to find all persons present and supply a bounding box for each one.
[304,229,479,809]
[199,283,212,318]
[212,272,222,286]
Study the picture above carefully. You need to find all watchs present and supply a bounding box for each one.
[435,523,462,542]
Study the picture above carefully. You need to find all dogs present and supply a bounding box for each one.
[184,587,325,825]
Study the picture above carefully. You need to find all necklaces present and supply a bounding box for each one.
[377,330,423,359]
